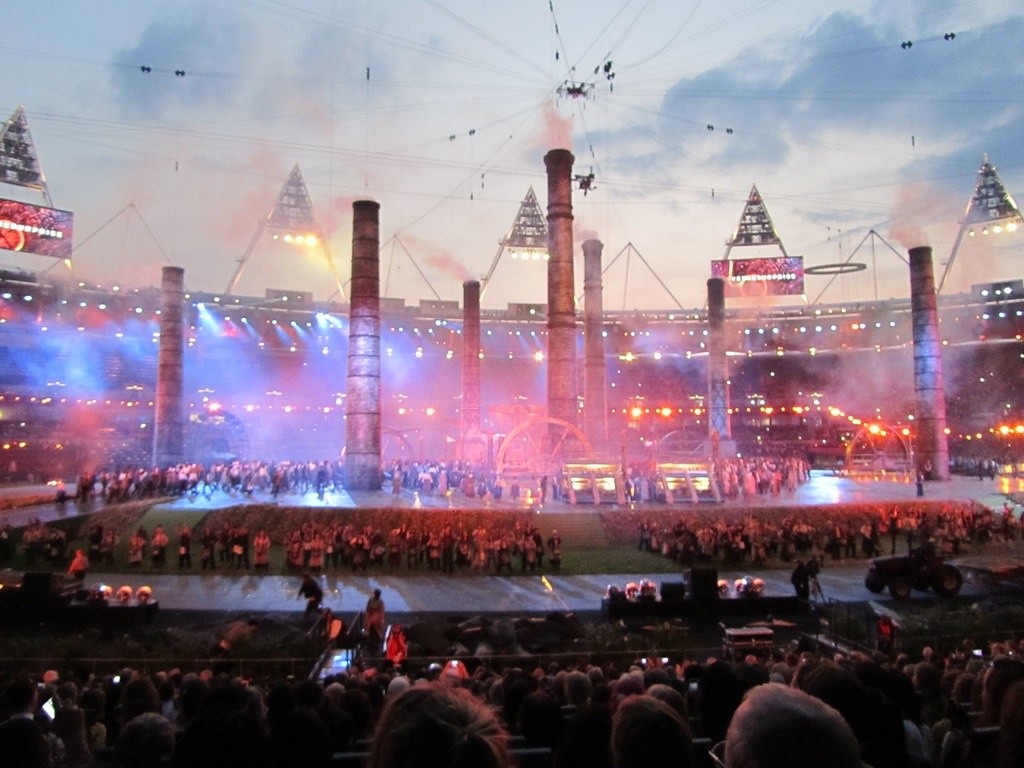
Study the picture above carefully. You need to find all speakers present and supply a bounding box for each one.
[683,569,719,600]
[22,571,78,607]
[660,582,685,600]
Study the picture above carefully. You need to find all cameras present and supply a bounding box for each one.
[662,657,669,663]
[641,658,646,664]
[973,650,983,656]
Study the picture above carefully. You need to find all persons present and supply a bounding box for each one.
[56,462,344,506]
[638,503,1024,599]
[710,428,812,509]
[948,456,1001,482]
[917,460,931,497]
[0,516,562,617]
[393,460,560,503]
[1,589,1023,768]
[622,468,664,504]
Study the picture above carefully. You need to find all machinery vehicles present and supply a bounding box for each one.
[864,547,964,602]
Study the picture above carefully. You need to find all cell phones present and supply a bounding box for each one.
[42,697,56,720]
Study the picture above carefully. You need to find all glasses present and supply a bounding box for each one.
[709,740,727,768]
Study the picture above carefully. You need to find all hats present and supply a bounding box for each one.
[327,683,346,702]
[629,670,645,689]
[386,677,410,692]
[922,646,934,656]
